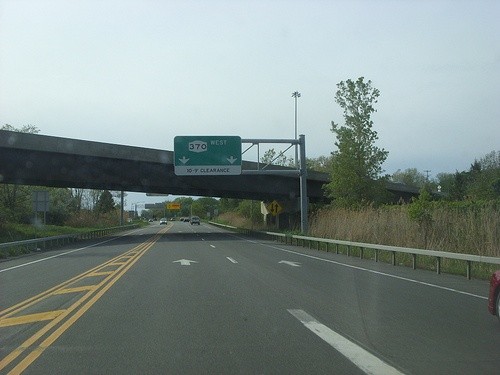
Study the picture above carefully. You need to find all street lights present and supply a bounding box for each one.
[291,92,301,169]
[424,170,431,182]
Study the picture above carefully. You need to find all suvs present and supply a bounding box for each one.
[190,216,200,225]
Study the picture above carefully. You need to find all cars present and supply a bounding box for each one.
[171,217,177,221]
[180,217,184,221]
[486,270,500,321]
[159,218,168,225]
[148,218,153,222]
[183,217,190,222]
